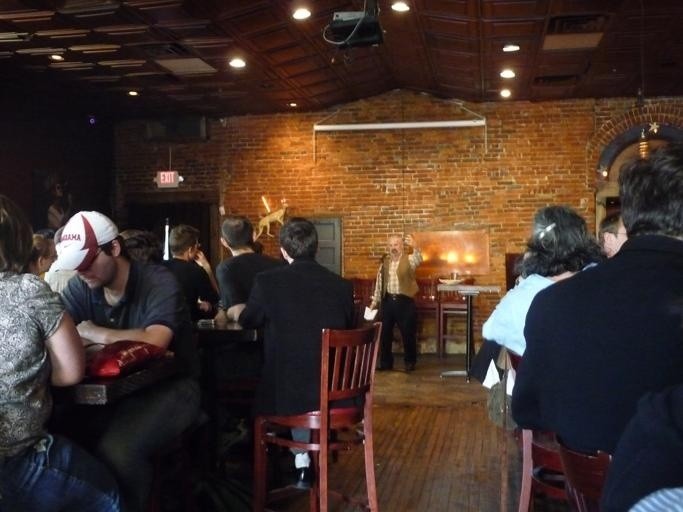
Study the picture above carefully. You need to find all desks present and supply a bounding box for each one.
[55,351,175,407]
[197,317,248,349]
[436,281,501,382]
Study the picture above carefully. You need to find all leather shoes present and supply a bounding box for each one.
[291,465,314,492]
[404,362,417,372]
[378,365,394,371]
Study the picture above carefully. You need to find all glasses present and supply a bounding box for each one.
[613,231,628,236]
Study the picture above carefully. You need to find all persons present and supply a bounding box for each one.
[597,211,628,259]
[0,194,123,511]
[46,184,72,230]
[599,383,683,509]
[119,229,161,265]
[482,207,604,357]
[214,218,354,489]
[511,142,683,509]
[369,235,423,372]
[26,234,57,280]
[212,216,285,323]
[53,212,202,511]
[162,225,219,320]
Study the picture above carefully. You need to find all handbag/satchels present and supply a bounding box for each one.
[487,377,519,431]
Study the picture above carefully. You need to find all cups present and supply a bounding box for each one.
[450,272,458,280]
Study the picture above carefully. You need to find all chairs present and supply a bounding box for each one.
[254,323,383,512]
[352,277,376,314]
[439,278,474,357]
[414,279,440,359]
[518,430,611,511]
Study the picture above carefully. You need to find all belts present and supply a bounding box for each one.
[384,293,413,301]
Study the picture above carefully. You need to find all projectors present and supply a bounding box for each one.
[329,0,384,50]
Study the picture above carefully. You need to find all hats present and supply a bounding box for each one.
[45,209,119,275]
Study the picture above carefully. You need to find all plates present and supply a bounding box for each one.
[439,280,464,285]
